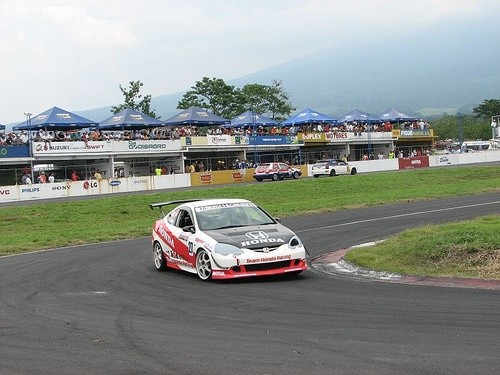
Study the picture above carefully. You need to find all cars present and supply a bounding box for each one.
[253,162,302,181]
[311,158,358,178]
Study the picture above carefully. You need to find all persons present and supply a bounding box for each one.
[0,118,500,186]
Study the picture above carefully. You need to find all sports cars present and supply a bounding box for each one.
[149,198,309,282]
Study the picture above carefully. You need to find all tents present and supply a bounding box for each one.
[282,106,338,126]
[162,105,227,127]
[97,107,164,131]
[336,109,382,125]
[377,108,422,123]
[13,105,102,132]
[225,111,278,128]
